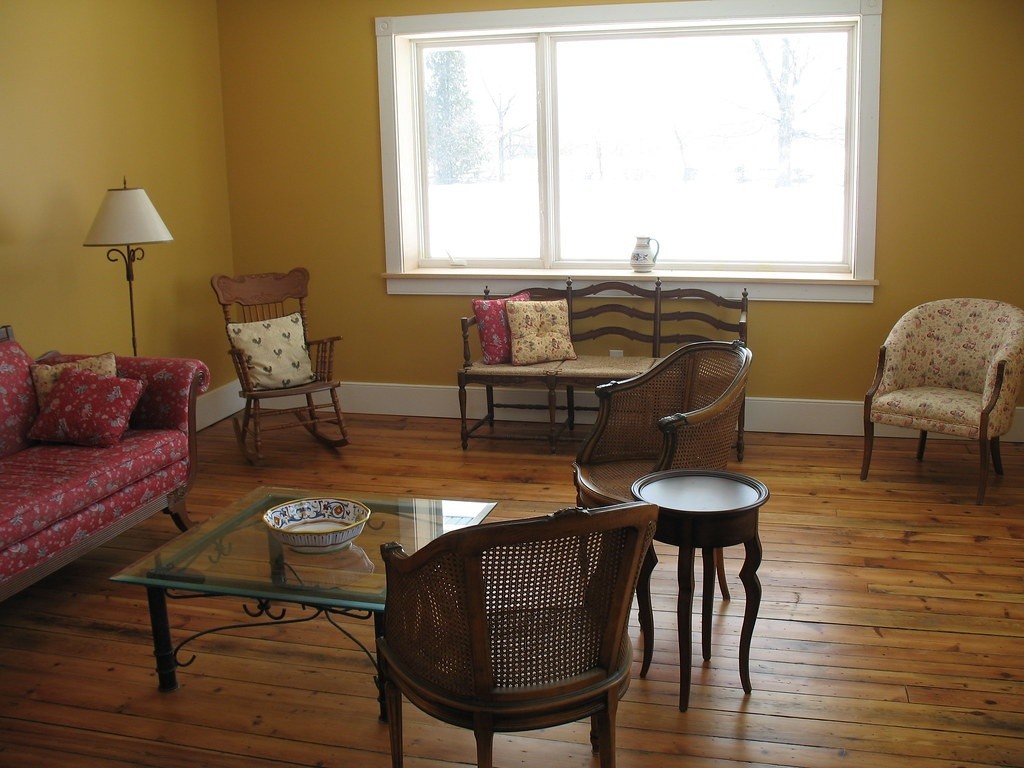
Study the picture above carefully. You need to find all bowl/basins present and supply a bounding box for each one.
[263,496,372,553]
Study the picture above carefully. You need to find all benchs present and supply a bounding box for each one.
[457,278,749,462]
[0,325,210,603]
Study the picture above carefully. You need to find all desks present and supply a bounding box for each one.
[631,467,770,713]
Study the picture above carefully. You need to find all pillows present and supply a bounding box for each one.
[28,352,129,431]
[226,311,319,392]
[27,365,144,449]
[505,299,577,366]
[471,291,531,365]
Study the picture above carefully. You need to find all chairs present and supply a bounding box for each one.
[861,298,1024,506]
[375,501,659,768]
[211,267,352,465]
[572,341,753,602]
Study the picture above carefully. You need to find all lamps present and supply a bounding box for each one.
[83,176,174,357]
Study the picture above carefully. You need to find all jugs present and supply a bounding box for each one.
[629,237,660,273]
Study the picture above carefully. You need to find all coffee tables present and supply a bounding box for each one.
[109,487,498,722]
